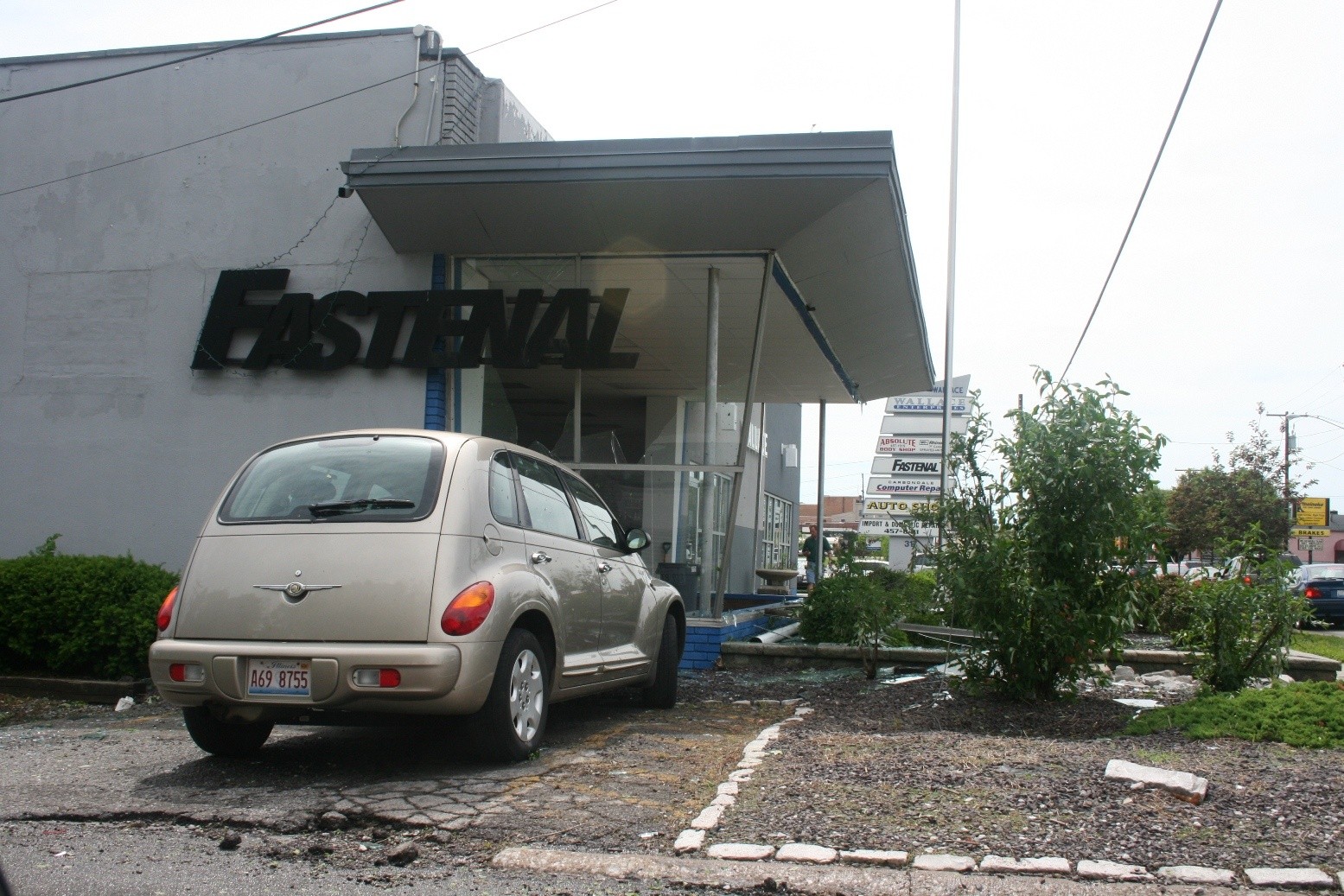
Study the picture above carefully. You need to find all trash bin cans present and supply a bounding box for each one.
[656,562,701,612]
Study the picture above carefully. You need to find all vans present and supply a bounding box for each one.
[847,560,890,576]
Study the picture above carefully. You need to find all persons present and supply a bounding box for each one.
[802,524,833,599]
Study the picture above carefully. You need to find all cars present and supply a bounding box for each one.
[152,426,689,767]
[1278,562,1344,631]
[797,558,810,589]
[1084,552,1303,591]
[905,552,944,578]
